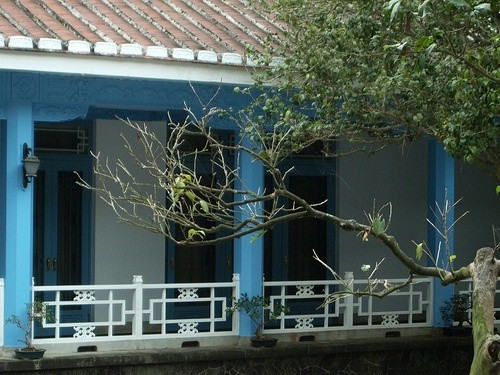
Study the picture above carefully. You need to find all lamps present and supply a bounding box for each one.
[19,142,39,189]
